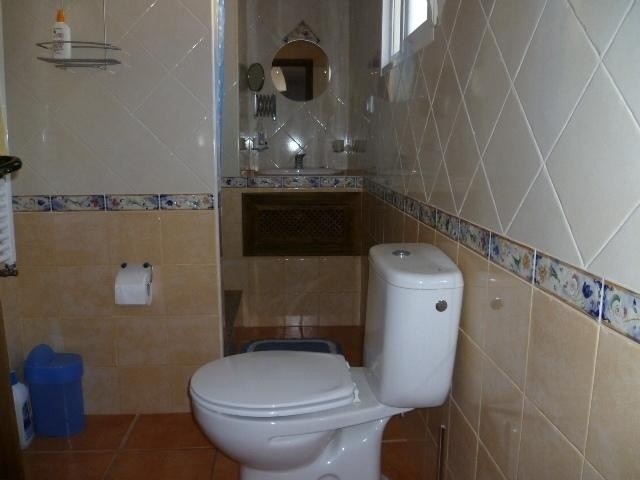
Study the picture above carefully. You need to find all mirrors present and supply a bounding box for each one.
[271,19,334,103]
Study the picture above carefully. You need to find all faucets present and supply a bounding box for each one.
[294,150,306,170]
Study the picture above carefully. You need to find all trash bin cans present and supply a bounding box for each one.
[247,339,333,354]
[25,343,84,437]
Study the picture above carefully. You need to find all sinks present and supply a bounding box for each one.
[256,168,338,176]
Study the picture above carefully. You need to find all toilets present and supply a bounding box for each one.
[188,240,463,479]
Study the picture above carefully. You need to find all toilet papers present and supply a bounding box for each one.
[114,272,153,305]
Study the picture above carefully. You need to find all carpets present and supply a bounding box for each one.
[242,337,341,357]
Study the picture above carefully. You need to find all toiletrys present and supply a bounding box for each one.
[254,118,266,148]
